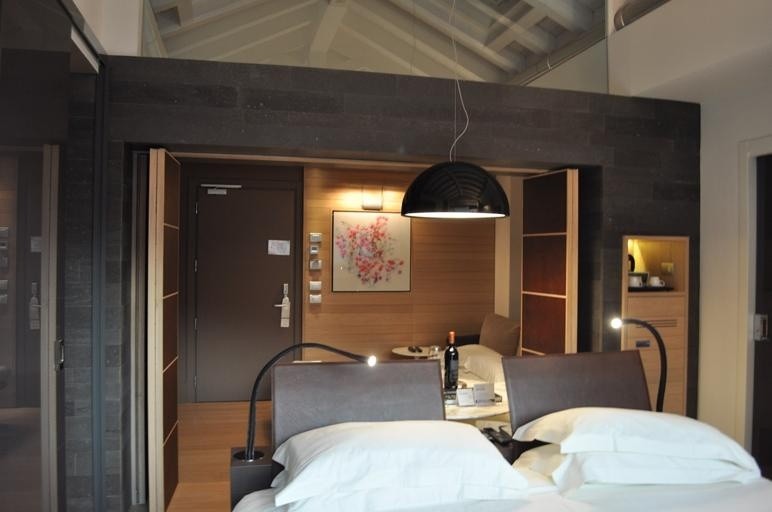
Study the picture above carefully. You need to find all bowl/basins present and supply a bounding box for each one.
[628,272,648,282]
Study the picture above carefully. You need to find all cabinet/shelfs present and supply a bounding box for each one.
[621,235,689,418]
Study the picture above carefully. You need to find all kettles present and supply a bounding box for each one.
[628,255,635,272]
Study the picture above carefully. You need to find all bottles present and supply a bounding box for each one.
[444,332,460,390]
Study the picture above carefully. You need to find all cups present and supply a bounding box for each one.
[428,345,443,360]
[630,276,642,287]
[649,277,665,287]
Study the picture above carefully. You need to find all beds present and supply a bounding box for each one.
[231,351,771,512]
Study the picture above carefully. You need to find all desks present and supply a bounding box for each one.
[443,392,510,429]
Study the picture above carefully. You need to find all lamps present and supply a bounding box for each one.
[610,317,668,412]
[363,197,384,209]
[403,0,511,220]
[230,342,376,512]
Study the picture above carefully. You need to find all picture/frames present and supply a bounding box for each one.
[332,210,411,292]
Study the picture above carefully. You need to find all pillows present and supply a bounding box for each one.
[511,406,761,474]
[271,419,528,507]
[270,469,530,512]
[443,343,506,382]
[513,444,757,497]
[480,313,518,357]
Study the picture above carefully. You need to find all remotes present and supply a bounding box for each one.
[487,427,512,445]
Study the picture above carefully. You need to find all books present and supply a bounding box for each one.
[443,392,503,405]
